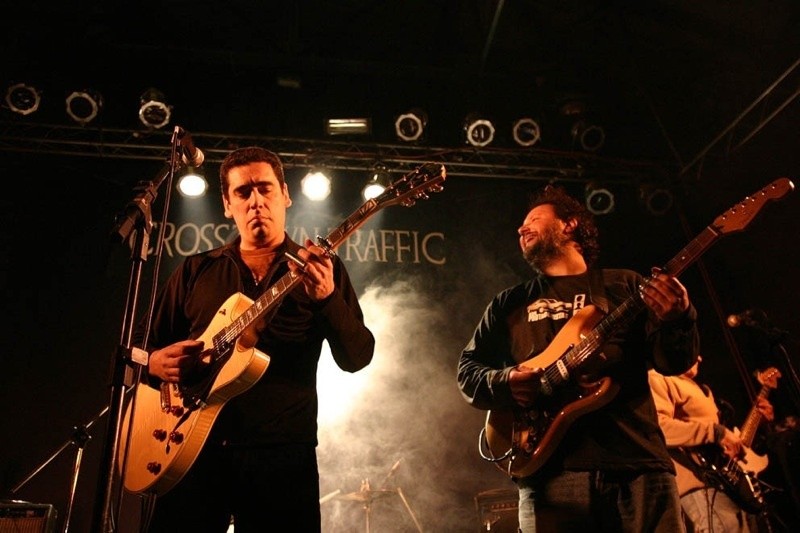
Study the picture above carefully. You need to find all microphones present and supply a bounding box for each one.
[176,126,204,167]
[380,459,401,486]
[727,309,754,328]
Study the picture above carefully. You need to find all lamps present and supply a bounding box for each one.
[175,160,210,199]
[298,164,333,203]
[4,76,171,129]
[322,115,373,140]
[360,162,392,201]
[393,107,676,217]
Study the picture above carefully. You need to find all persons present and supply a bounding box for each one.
[130,146,375,533]
[456,191,700,533]
[647,354,774,533]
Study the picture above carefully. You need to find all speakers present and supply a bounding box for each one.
[474,489,521,533]
[0,499,58,533]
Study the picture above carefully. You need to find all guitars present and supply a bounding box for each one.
[116,161,447,498]
[485,173,795,478]
[694,364,782,510]
